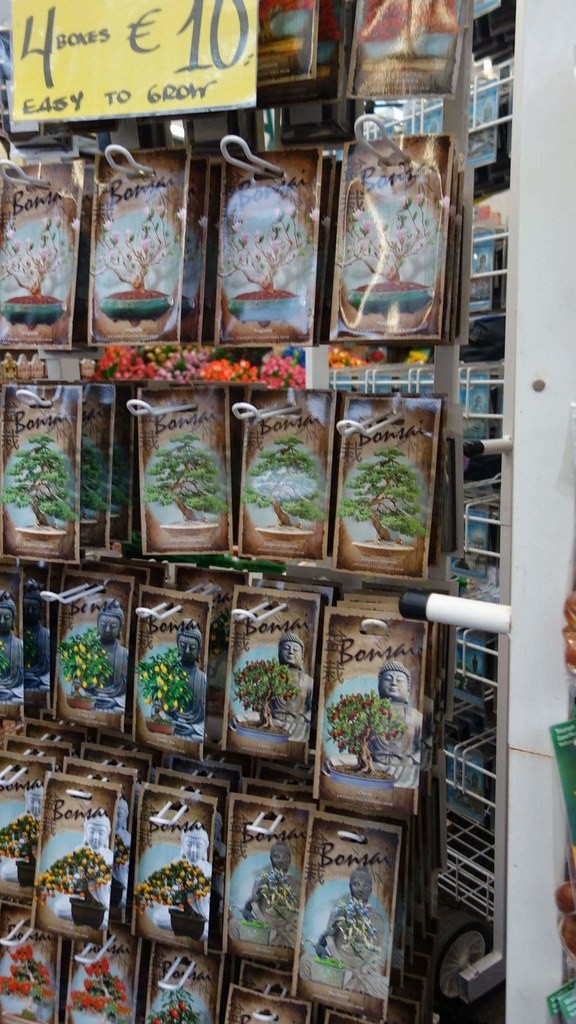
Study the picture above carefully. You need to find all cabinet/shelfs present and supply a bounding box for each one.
[0,1,576,1024]
